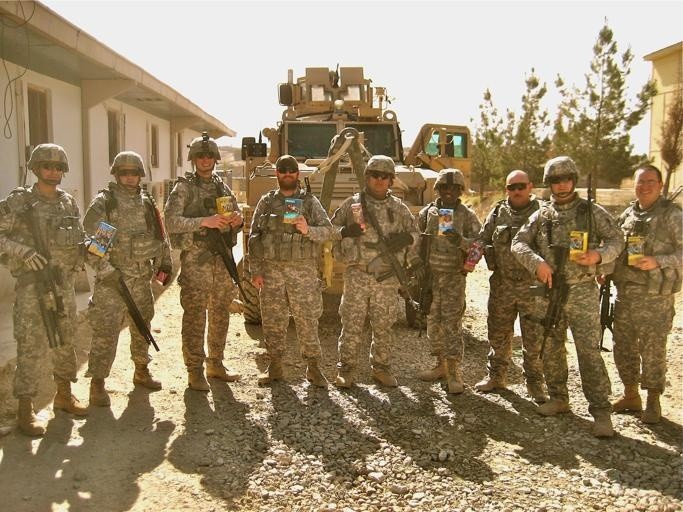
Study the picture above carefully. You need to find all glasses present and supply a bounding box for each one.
[196,152,214,160]
[41,161,64,172]
[277,167,297,174]
[508,183,529,191]
[120,169,139,177]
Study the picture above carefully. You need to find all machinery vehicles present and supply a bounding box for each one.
[235,63,474,326]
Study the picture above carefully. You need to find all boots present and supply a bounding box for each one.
[132,364,161,389]
[188,368,210,390]
[305,359,328,387]
[207,363,239,380]
[526,382,571,415]
[418,356,465,394]
[475,376,506,393]
[89,378,112,407]
[335,372,352,388]
[372,365,398,387]
[593,387,662,436]
[256,354,281,383]
[53,385,86,417]
[16,396,47,437]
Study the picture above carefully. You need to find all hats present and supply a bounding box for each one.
[363,155,395,178]
[273,154,299,169]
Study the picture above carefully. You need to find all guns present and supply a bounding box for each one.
[365,210,409,286]
[193,197,250,305]
[95,267,159,351]
[17,207,68,350]
[600,273,614,351]
[524,243,570,360]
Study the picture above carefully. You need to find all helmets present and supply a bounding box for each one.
[544,156,578,180]
[110,151,147,177]
[27,143,70,172]
[433,169,465,191]
[188,136,222,161]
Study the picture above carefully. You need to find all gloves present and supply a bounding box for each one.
[409,255,425,279]
[157,264,172,286]
[386,232,413,252]
[15,244,47,271]
[343,223,365,238]
[446,230,461,241]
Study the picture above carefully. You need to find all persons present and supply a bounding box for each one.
[414,167,482,395]
[329,154,420,389]
[160,130,246,393]
[507,154,625,436]
[80,150,172,410]
[0,143,89,437]
[595,164,682,425]
[474,169,550,404]
[247,153,336,392]
